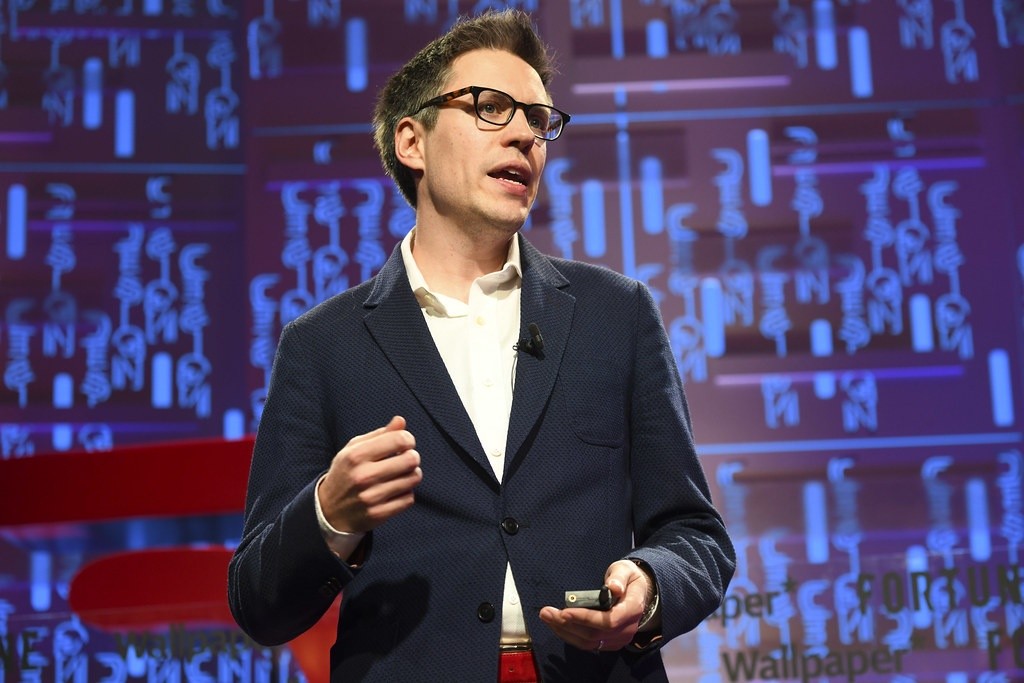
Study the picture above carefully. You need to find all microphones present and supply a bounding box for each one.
[528,323,545,350]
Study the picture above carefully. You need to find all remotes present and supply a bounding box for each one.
[563,586,613,610]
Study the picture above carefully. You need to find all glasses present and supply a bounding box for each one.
[408,85,570,141]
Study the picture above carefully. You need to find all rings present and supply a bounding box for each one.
[597,640,605,650]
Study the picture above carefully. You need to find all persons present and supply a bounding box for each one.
[225,8,736,683]
